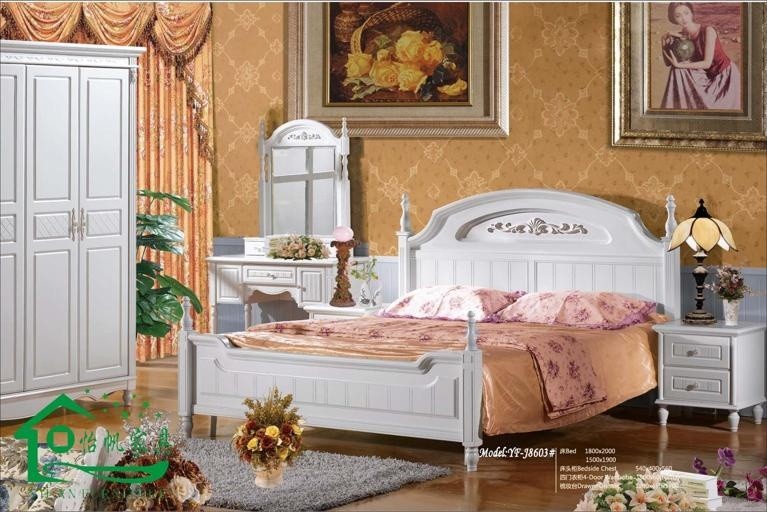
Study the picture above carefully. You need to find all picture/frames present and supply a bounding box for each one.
[282,3,510,139]
[611,1,767,154]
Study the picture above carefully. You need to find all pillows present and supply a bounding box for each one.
[492,290,656,330]
[375,286,528,322]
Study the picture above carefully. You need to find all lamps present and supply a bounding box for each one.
[668,198,740,325]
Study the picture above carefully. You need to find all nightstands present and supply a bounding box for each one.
[651,319,767,433]
[301,305,379,322]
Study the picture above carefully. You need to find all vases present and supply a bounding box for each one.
[722,299,740,326]
[254,459,284,489]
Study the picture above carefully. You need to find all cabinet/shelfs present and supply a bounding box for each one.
[0,40,150,424]
[205,253,370,334]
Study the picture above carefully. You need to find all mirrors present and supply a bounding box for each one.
[258,115,354,257]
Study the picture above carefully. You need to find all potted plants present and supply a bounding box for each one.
[351,260,380,308]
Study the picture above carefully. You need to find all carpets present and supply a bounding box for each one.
[99,435,449,512]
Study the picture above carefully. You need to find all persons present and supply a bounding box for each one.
[660,2,740,110]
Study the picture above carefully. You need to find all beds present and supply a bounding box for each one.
[178,188,681,473]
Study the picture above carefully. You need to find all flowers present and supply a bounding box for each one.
[269,235,330,261]
[232,384,305,478]
[574,467,704,512]
[711,264,753,303]
[692,446,767,502]
[97,448,212,512]
